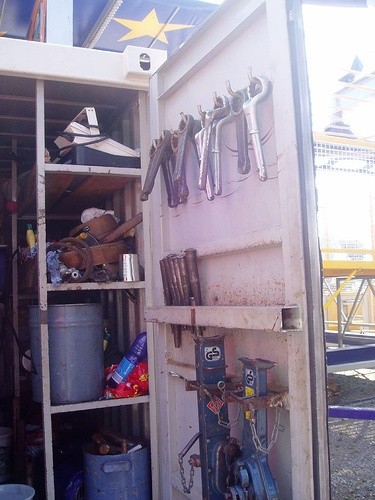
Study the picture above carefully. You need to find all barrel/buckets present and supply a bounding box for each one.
[26,302,104,405]
[0,427,13,485]
[83,436,153,500]
[0,484,36,500]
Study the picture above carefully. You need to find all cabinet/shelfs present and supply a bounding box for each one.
[7,79,162,499]
